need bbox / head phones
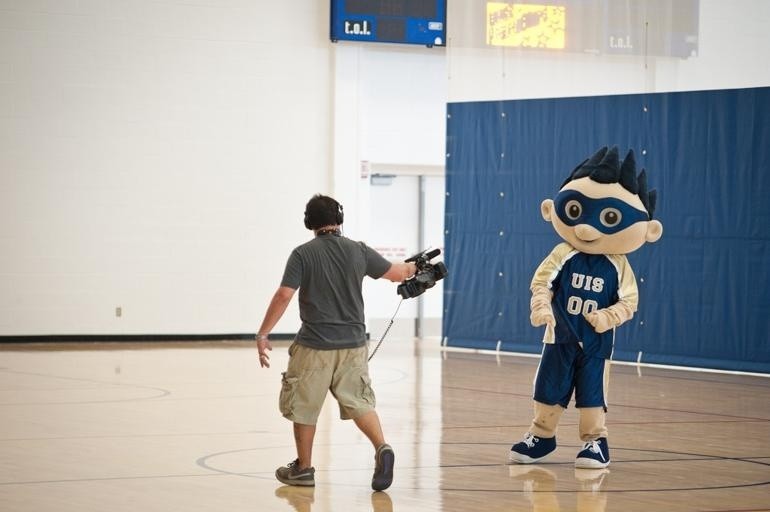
[304,203,344,230]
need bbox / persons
[275,485,393,512]
[255,192,419,491]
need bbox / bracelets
[256,336,268,339]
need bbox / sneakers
[371,443,395,491]
[275,460,316,486]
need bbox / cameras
[392,248,449,299]
